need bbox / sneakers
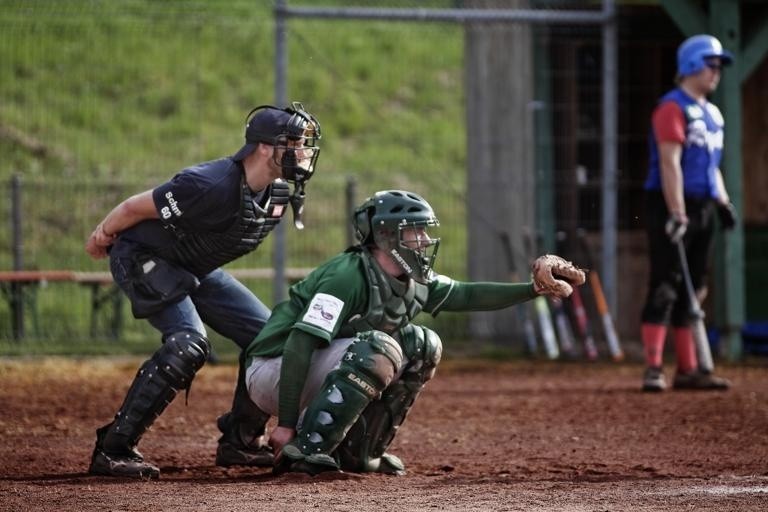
[214,438,274,467]
[91,428,160,481]
[674,370,731,388]
[642,365,667,389]
[276,448,407,476]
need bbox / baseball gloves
[531,252,587,304]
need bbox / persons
[243,189,585,474]
[85,101,321,481]
[640,34,737,391]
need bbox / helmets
[232,99,322,183]
[678,34,733,76]
[353,188,442,284]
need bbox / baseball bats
[496,227,625,366]
[670,214,715,377]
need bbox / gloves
[717,200,737,232]
[665,217,691,243]
